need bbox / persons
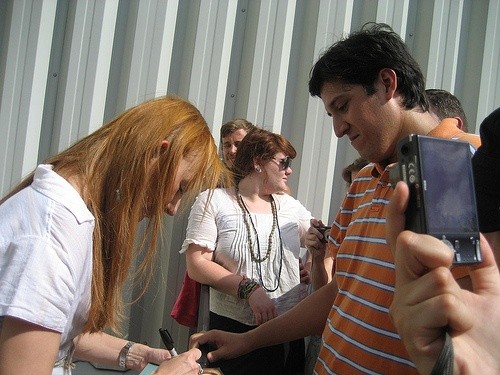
[0,96,204,375]
[180,127,315,375]
[341,90,500,375]
[220,120,293,196]
[188,22,482,375]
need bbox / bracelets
[118,341,135,372]
[236,276,259,299]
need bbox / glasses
[272,156,292,170]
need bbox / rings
[198,363,203,375]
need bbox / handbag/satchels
[170,270,200,327]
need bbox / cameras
[388,133,482,266]
[315,227,332,243]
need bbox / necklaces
[234,184,284,292]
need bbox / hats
[471,107,500,233]
